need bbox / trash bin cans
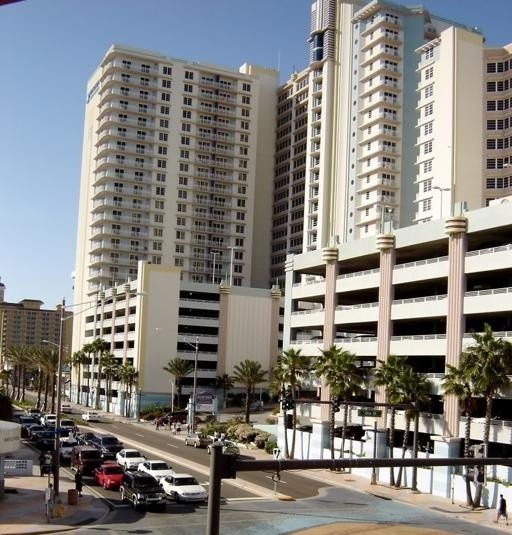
[68,489,78,505]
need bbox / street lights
[226,246,237,286]
[432,185,452,219]
[210,252,219,284]
[52,289,147,496]
[155,326,199,434]
[41,339,70,402]
[376,203,391,233]
[503,163,512,168]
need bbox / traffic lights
[283,390,295,410]
[334,396,340,412]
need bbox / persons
[50,483,55,518]
[38,450,52,476]
[75,469,84,497]
[154,414,191,436]
[493,494,508,526]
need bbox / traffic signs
[358,409,381,417]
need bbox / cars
[206,441,240,458]
[15,404,208,511]
[185,433,213,447]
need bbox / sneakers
[79,494,83,497]
[494,520,509,526]
